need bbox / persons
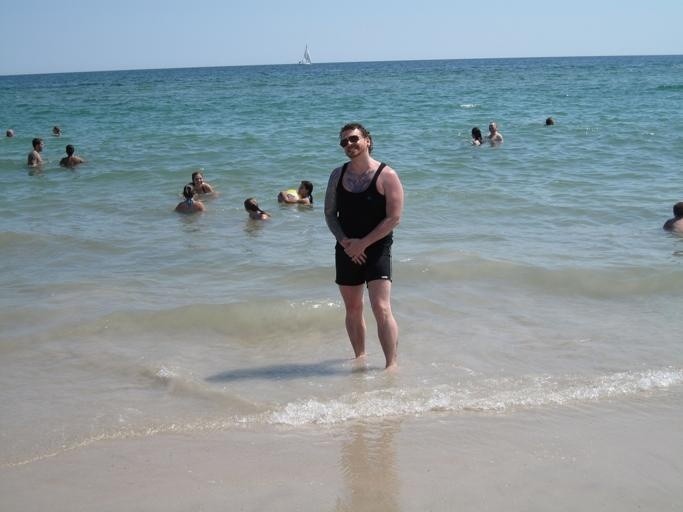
[488,122,502,144]
[663,202,683,231]
[279,181,313,205]
[471,127,483,146]
[191,172,212,194]
[6,129,14,137]
[176,185,204,212]
[244,198,270,219]
[546,116,555,124]
[324,124,404,368]
[60,145,84,166]
[53,126,61,136]
[27,138,43,165]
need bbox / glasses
[339,135,360,147]
